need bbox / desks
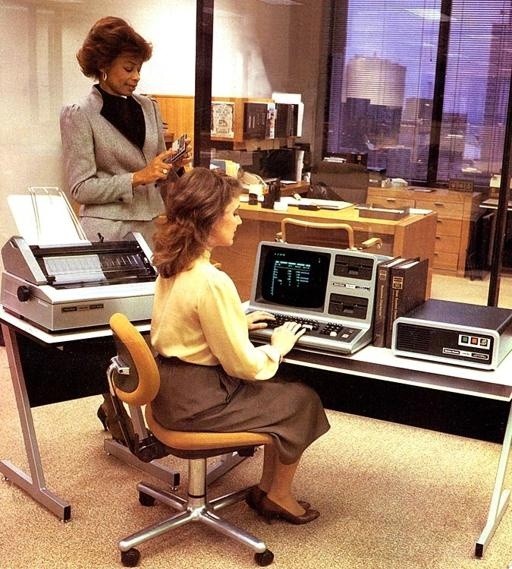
[204,200,436,311]
[0,308,512,557]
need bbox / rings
[162,168,168,175]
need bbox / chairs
[97,311,283,568]
[278,217,384,254]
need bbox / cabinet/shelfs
[209,96,312,198]
[364,187,482,276]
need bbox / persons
[58,14,193,435]
[139,165,339,526]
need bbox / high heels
[247,486,320,524]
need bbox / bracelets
[131,172,136,184]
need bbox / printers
[1,230,159,333]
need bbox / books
[207,90,313,199]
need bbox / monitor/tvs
[254,245,331,310]
